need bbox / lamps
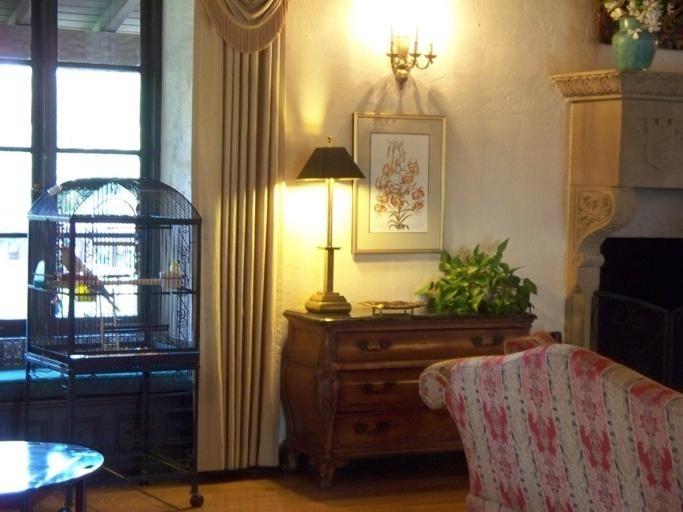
[386,14,438,82]
[296,136,366,313]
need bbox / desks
[0,438,104,511]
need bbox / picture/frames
[352,111,447,255]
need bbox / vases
[609,17,657,69]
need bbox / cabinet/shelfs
[275,310,539,493]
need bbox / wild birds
[56,243,122,312]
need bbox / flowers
[601,0,672,42]
